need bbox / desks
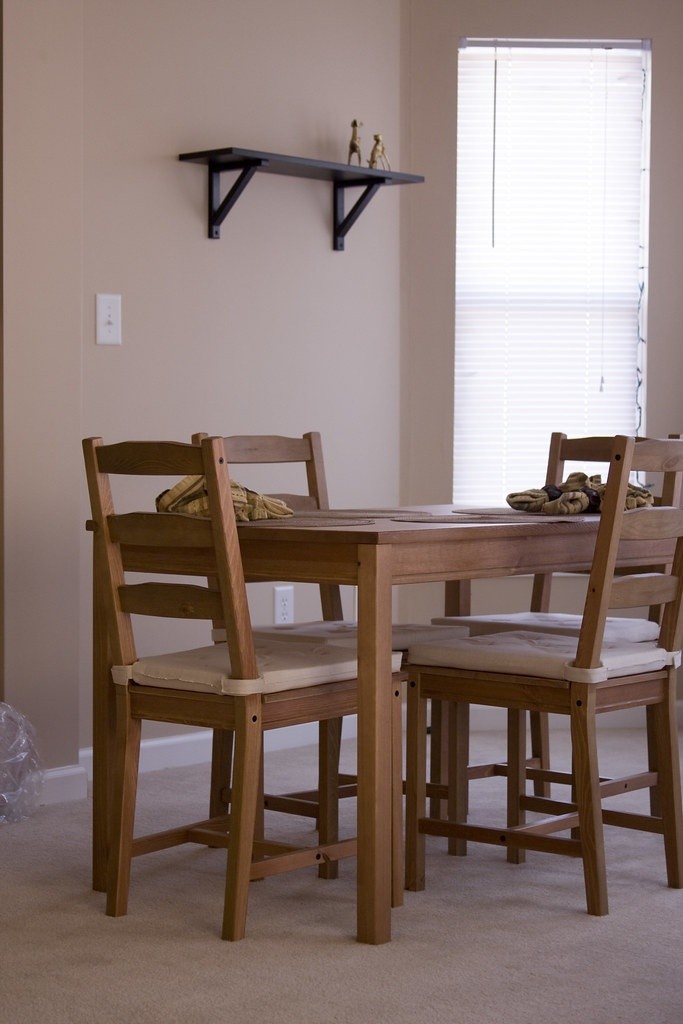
[81,507,678,945]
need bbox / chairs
[428,432,680,857]
[82,433,412,942]
[404,435,683,916]
[188,433,471,879]
